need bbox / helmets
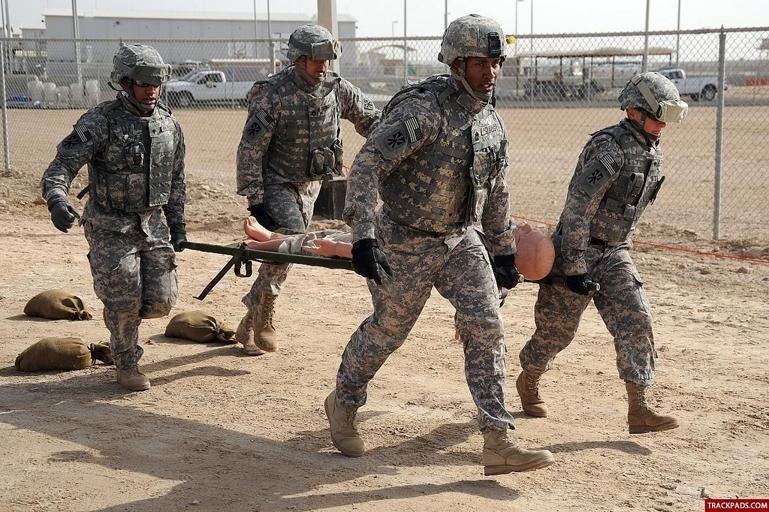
[287,24,342,63]
[437,13,508,65]
[618,72,688,122]
[109,42,171,87]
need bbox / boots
[235,311,264,354]
[324,389,364,457]
[626,382,679,433]
[482,431,554,476]
[252,291,278,352]
[515,364,547,418]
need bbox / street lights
[391,21,398,66]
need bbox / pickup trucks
[658,69,728,103]
[165,71,258,106]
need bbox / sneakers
[117,364,150,391]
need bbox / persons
[244,215,556,281]
[515,71,688,434]
[40,44,188,390]
[323,13,556,475]
[234,24,382,357]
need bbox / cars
[6,95,41,108]
[561,76,597,99]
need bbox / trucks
[168,59,282,84]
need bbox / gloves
[247,203,281,231]
[47,194,79,233]
[170,232,187,252]
[494,255,520,289]
[351,239,393,285]
[567,274,593,295]
